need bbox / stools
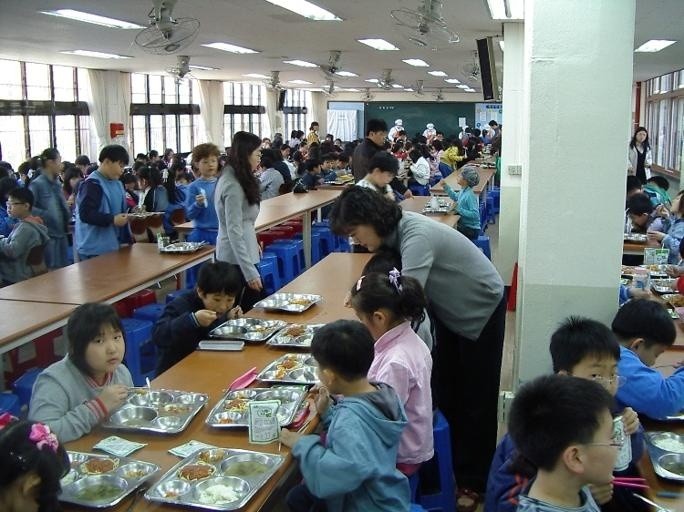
[479,202,488,231]
[487,198,495,223]
[265,243,301,284]
[127,289,157,309]
[0,392,22,417]
[112,300,134,317]
[261,252,277,266]
[256,260,281,293]
[294,233,321,265]
[12,367,46,411]
[166,288,192,306]
[260,231,287,245]
[134,302,168,322]
[274,239,306,272]
[507,262,518,311]
[492,190,499,213]
[121,318,157,386]
[409,410,457,512]
[270,226,295,239]
[313,222,329,227]
[282,221,302,232]
[312,227,334,253]
[470,236,491,260]
[289,215,303,220]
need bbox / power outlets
[517,165,522,174]
[508,165,517,174]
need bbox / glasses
[5,201,26,207]
[583,431,625,451]
[593,375,626,389]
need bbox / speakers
[477,37,500,101]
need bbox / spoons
[144,377,159,404]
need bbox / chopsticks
[290,418,318,437]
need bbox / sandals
[456,489,478,511]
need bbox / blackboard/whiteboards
[365,101,475,140]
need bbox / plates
[101,387,211,435]
[263,322,328,348]
[206,315,287,342]
[204,384,310,428]
[424,194,453,216]
[129,207,168,223]
[56,450,160,509]
[143,446,288,512]
[643,429,684,481]
[253,290,324,313]
[255,350,321,384]
[649,260,684,292]
[159,243,208,253]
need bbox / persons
[480,129,491,144]
[319,154,338,183]
[281,146,291,158]
[335,153,349,177]
[189,159,202,181]
[441,167,481,240]
[430,140,444,164]
[154,262,245,373]
[668,237,684,294]
[329,185,504,453]
[440,137,464,169]
[165,158,186,205]
[145,150,160,163]
[290,130,304,148]
[0,160,13,177]
[468,128,484,151]
[18,162,31,183]
[176,172,195,185]
[628,194,666,234]
[214,131,262,313]
[259,156,284,202]
[347,150,399,252]
[261,137,282,160]
[136,166,169,212]
[74,155,91,175]
[183,143,218,245]
[1,188,50,288]
[85,165,99,178]
[359,252,434,354]
[62,166,84,210]
[396,130,407,143]
[155,160,168,183]
[307,120,321,145]
[649,190,684,263]
[28,302,134,444]
[294,142,308,161]
[162,148,175,166]
[0,177,25,239]
[322,141,335,160]
[388,119,404,144]
[508,375,619,512]
[487,319,644,512]
[271,150,293,195]
[619,285,650,310]
[276,320,410,512]
[489,120,501,156]
[330,153,339,171]
[410,136,429,164]
[293,159,324,193]
[27,148,72,273]
[390,143,408,161]
[132,160,144,177]
[628,127,654,181]
[74,145,134,263]
[432,132,448,151]
[408,150,431,186]
[0,413,72,512]
[298,146,324,174]
[350,271,434,475]
[325,134,333,141]
[335,138,345,150]
[123,172,141,208]
[612,298,684,418]
[262,138,271,149]
[136,153,146,164]
[457,140,478,168]
[627,175,668,211]
[294,150,305,179]
[350,120,387,183]
[422,123,436,145]
[416,145,441,178]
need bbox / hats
[463,168,479,186]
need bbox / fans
[432,88,444,102]
[134,1,201,56]
[362,88,376,102]
[322,81,338,98]
[262,70,286,93]
[319,50,350,82]
[460,49,481,83]
[376,69,396,91]
[166,55,199,84]
[411,80,426,98]
[390,1,460,49]
